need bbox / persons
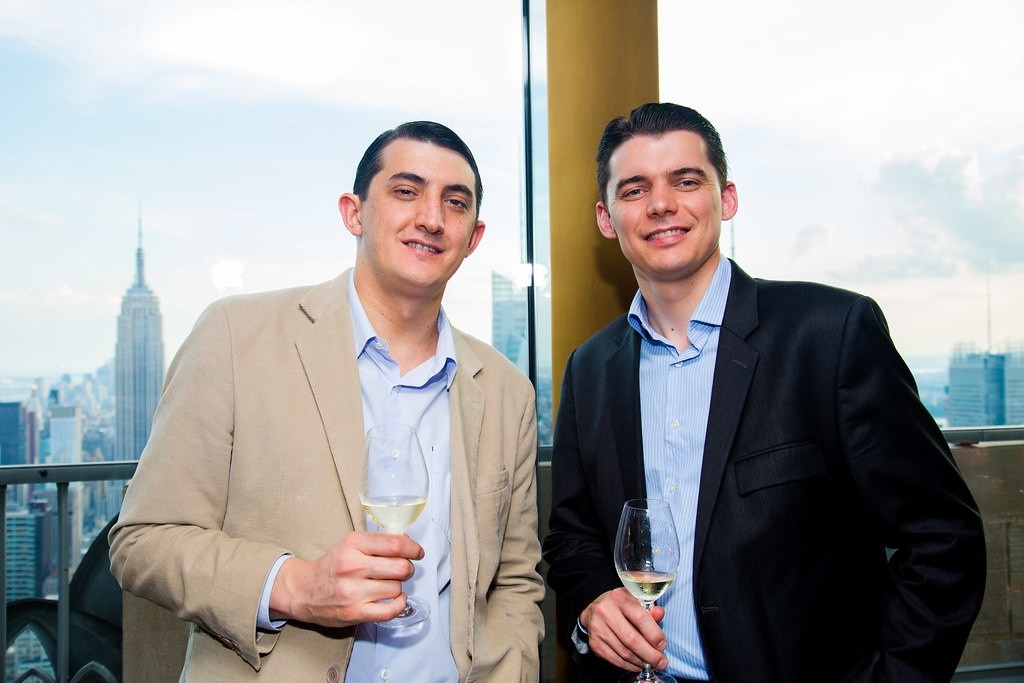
[540,102,987,683]
[106,120,545,683]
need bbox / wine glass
[359,427,434,628]
[612,498,682,683]
